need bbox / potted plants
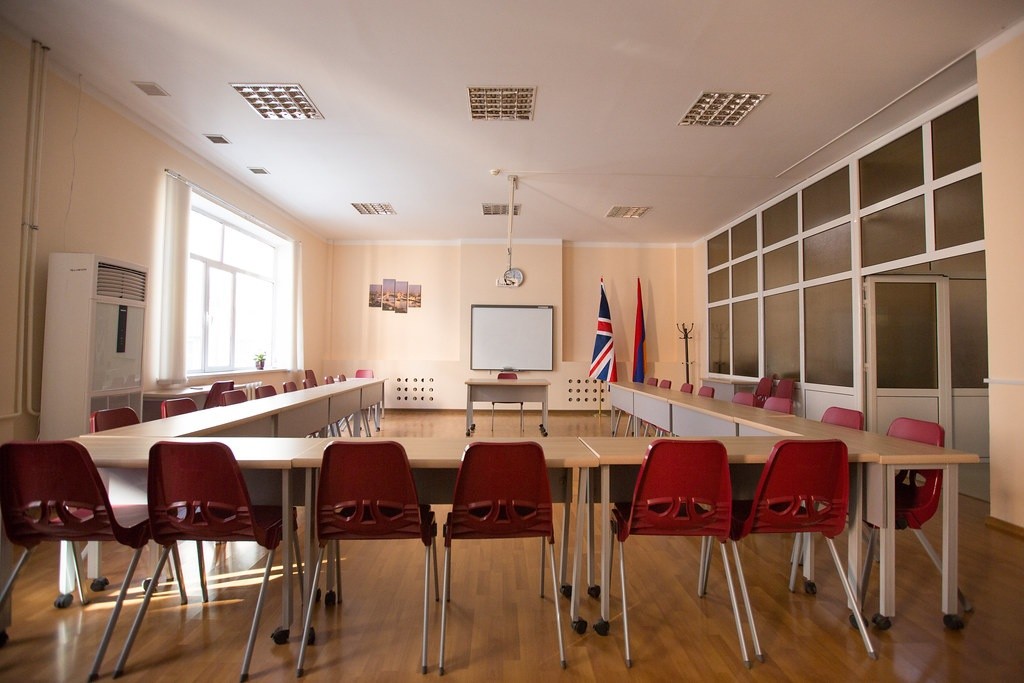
[252,353,266,370]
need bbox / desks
[59,392,980,560]
[141,384,249,423]
[293,437,600,645]
[463,378,550,436]
[607,382,657,436]
[333,377,377,384]
[58,436,321,643]
[700,378,758,404]
[338,377,388,433]
[579,436,882,633]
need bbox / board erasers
[502,367,512,371]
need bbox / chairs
[800,415,974,626]
[0,440,171,683]
[86,408,176,583]
[704,439,877,663]
[114,439,303,683]
[297,441,438,679]
[738,407,865,566]
[88,370,794,438]
[440,441,565,674]
[607,439,751,671]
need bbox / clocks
[504,269,524,287]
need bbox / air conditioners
[40,250,151,444]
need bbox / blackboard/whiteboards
[470,304,554,371]
